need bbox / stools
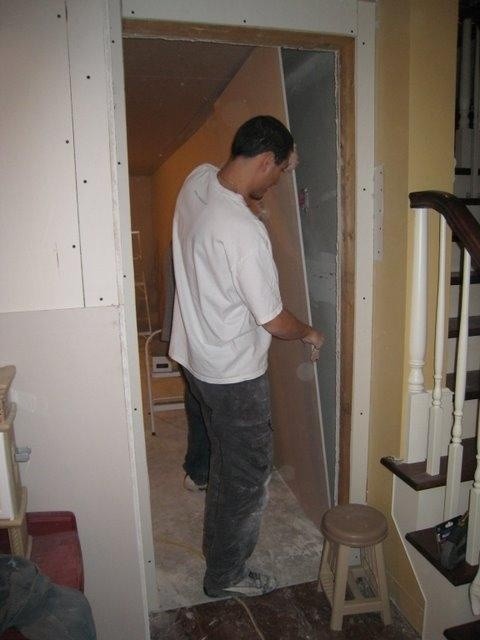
[314,503,393,632]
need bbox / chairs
[144,327,188,435]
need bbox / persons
[165,114,329,601]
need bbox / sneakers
[203,567,280,599]
[181,470,209,491]
